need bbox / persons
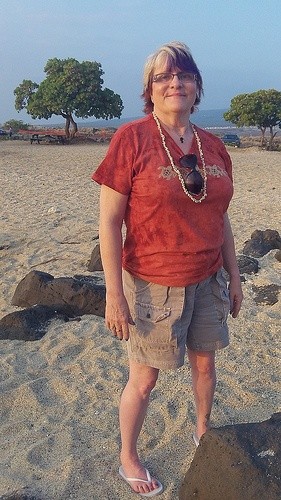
[90,40,244,499]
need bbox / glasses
[179,154,202,195]
[153,72,197,82]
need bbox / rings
[115,329,122,333]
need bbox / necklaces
[152,112,208,204]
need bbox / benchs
[30,139,66,145]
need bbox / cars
[0,129,24,140]
[221,134,241,147]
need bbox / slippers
[193,433,200,447]
[119,464,163,496]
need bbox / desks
[32,134,66,144]
